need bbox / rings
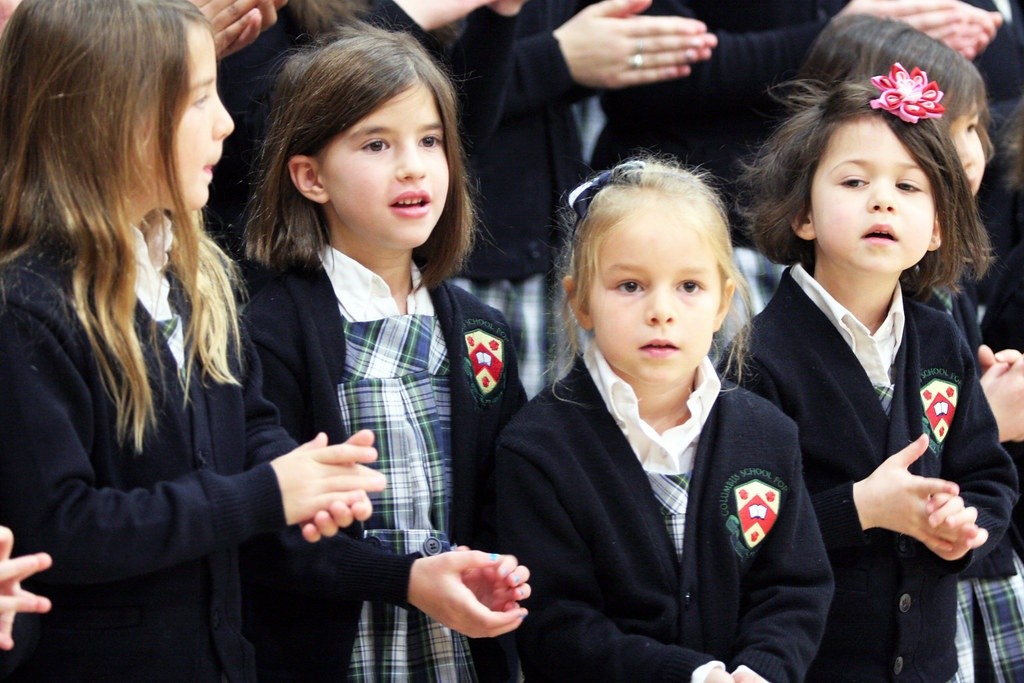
[629,52,644,69]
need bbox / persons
[490,150,837,683]
[783,11,1023,681]
[194,2,1023,404]
[236,25,528,683]
[0,1,389,683]
[724,58,1020,681]
[0,523,51,652]
